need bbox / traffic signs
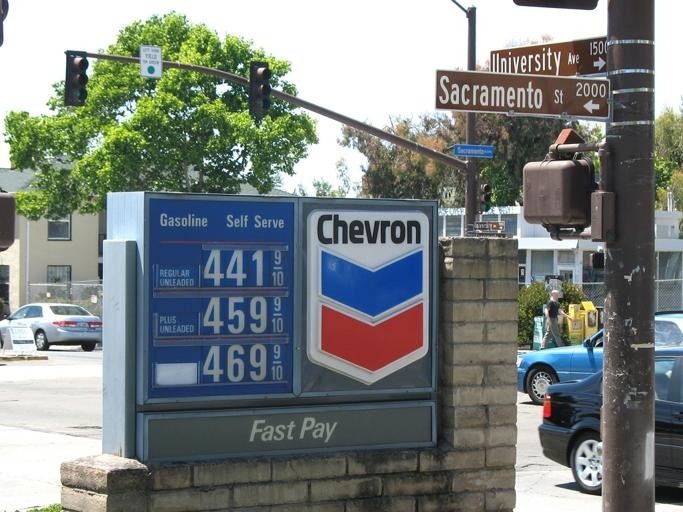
[477,232,506,238]
[139,45,163,79]
[454,144,495,158]
[435,70,609,125]
[487,36,607,78]
[473,220,505,230]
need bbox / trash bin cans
[533,301,603,350]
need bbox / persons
[539,290,573,349]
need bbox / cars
[515,310,682,406]
[0,303,102,351]
[539,346,682,498]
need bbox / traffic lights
[247,60,271,117]
[593,254,604,269]
[480,184,491,213]
[64,52,88,106]
